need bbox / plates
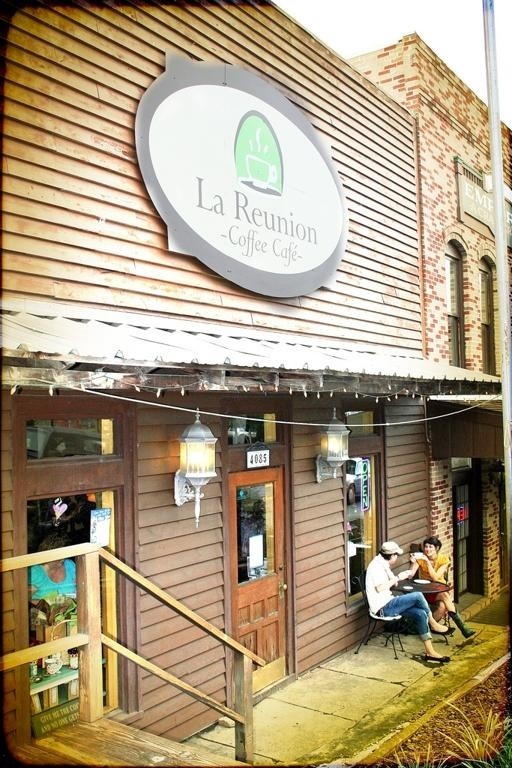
[413,579,431,584]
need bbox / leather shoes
[424,655,450,662]
[431,627,455,635]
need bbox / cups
[44,659,63,675]
[411,552,424,560]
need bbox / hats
[382,541,404,555]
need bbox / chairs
[351,550,455,660]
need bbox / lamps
[174,406,218,529]
[320,406,351,479]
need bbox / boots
[448,609,475,638]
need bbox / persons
[26,533,80,603]
[408,536,476,638]
[365,540,454,662]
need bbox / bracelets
[395,575,401,581]
[425,559,429,563]
